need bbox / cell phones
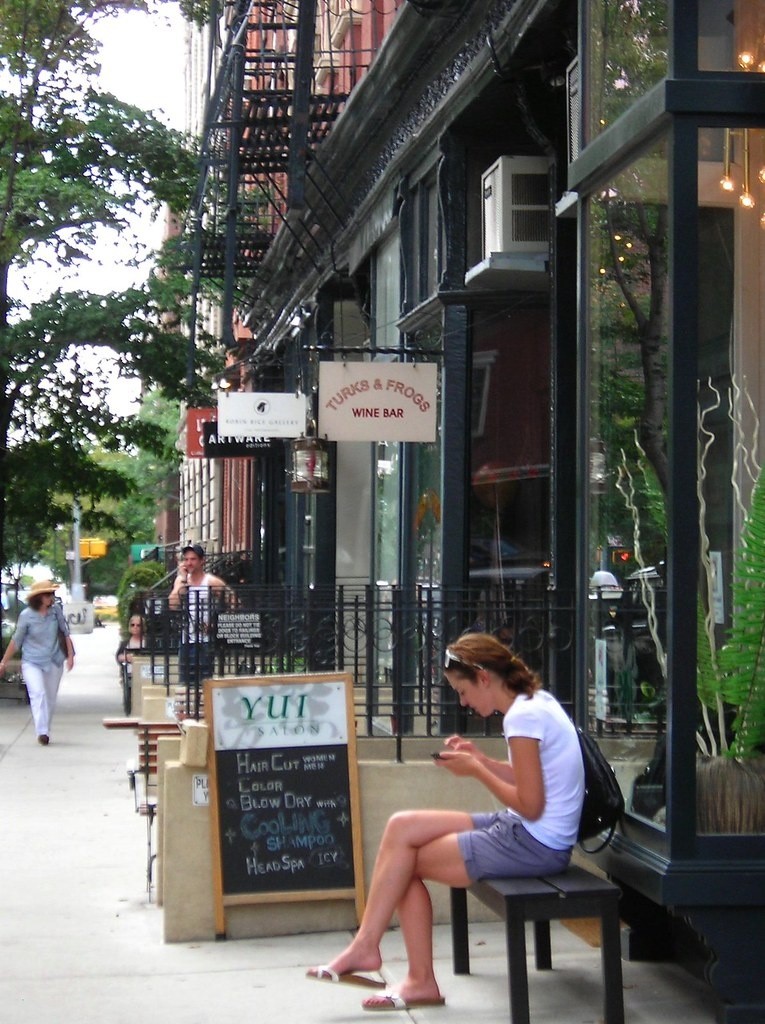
[431,752,444,761]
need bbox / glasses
[444,649,484,670]
[130,624,140,628]
[37,592,55,597]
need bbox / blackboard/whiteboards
[199,673,368,909]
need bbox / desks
[101,717,220,902]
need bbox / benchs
[451,864,626,1024]
[127,721,204,902]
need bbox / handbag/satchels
[574,722,625,854]
[53,605,75,658]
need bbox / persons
[306,632,585,1013]
[168,545,242,689]
[0,579,76,745]
[94,614,103,627]
[115,615,150,716]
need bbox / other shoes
[36,734,50,746]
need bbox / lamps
[737,50,765,72]
[719,128,765,231]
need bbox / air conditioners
[481,156,558,261]
[566,55,579,167]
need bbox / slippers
[304,964,386,984]
[361,989,446,1009]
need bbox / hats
[181,543,204,557]
[25,579,60,601]
